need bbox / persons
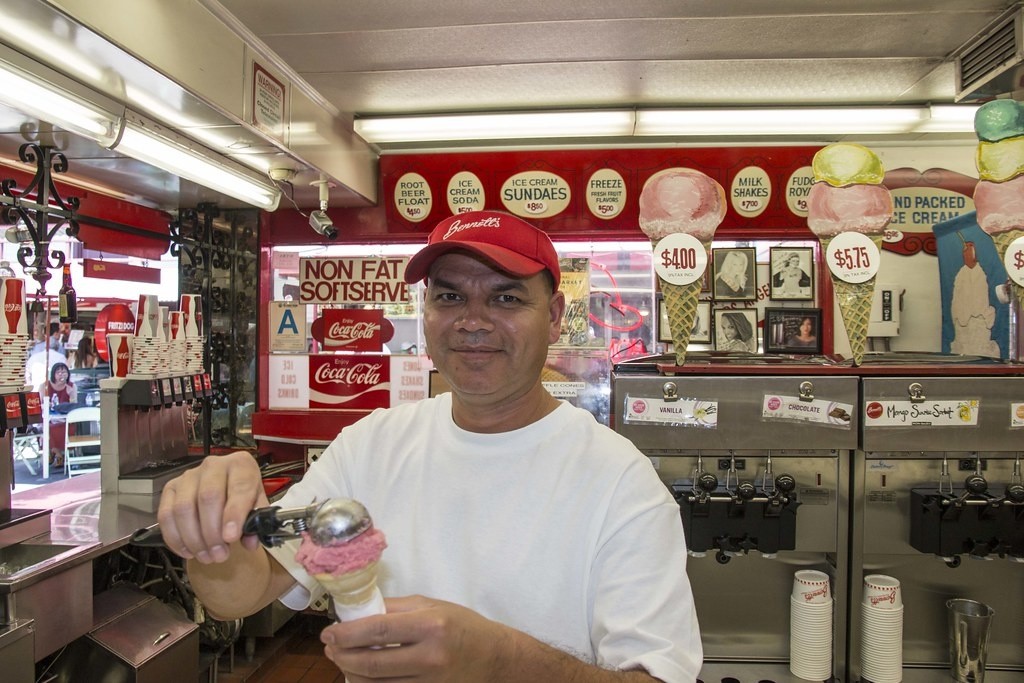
[788,317,816,346]
[773,252,811,295]
[718,313,754,352]
[24,323,99,469]
[156,211,703,683]
[715,250,748,296]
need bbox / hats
[404,210,560,295]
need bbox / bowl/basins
[860,574,903,683]
[789,570,833,681]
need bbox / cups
[946,598,995,683]
[104,293,204,379]
[0,277,31,394]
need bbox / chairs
[65,406,102,478]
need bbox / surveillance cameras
[309,210,337,240]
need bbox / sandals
[55,454,64,467]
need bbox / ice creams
[294,522,388,608]
[639,167,727,365]
[807,142,893,367]
[973,99,1024,309]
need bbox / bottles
[58,263,79,324]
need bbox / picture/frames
[712,309,757,353]
[657,298,712,344]
[764,307,823,353]
[712,247,758,300]
[770,247,814,299]
[651,243,711,293]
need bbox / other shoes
[49,451,56,464]
